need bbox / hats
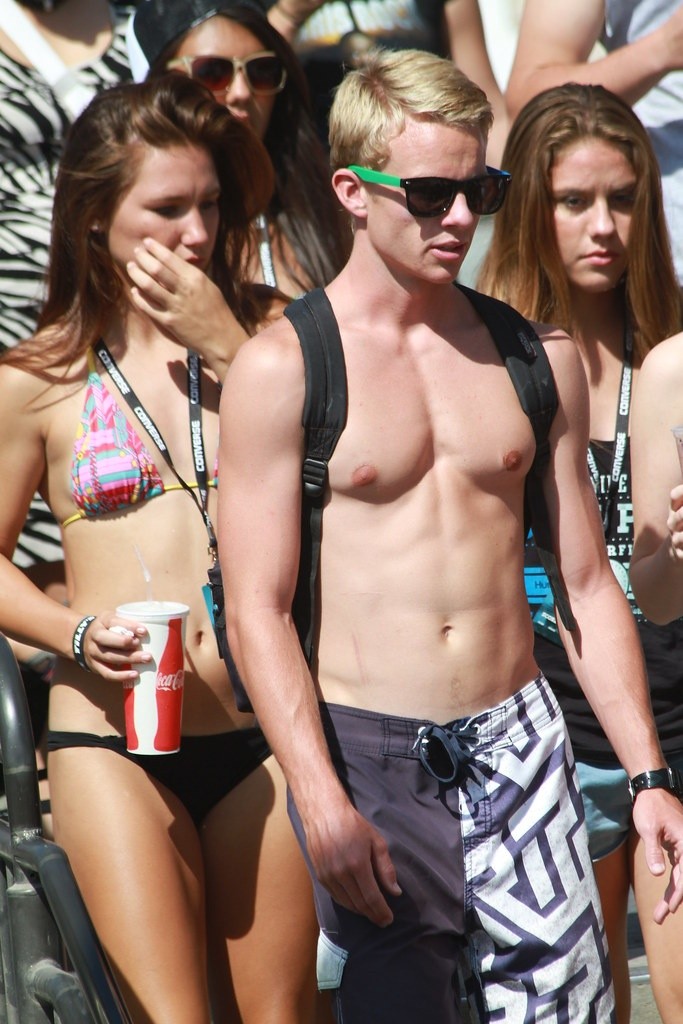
[133,0,227,82]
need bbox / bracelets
[73,616,97,675]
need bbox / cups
[115,600,190,756]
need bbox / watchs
[627,766,683,806]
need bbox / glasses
[348,165,511,219]
[167,52,288,96]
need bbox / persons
[0,0,683,1024]
